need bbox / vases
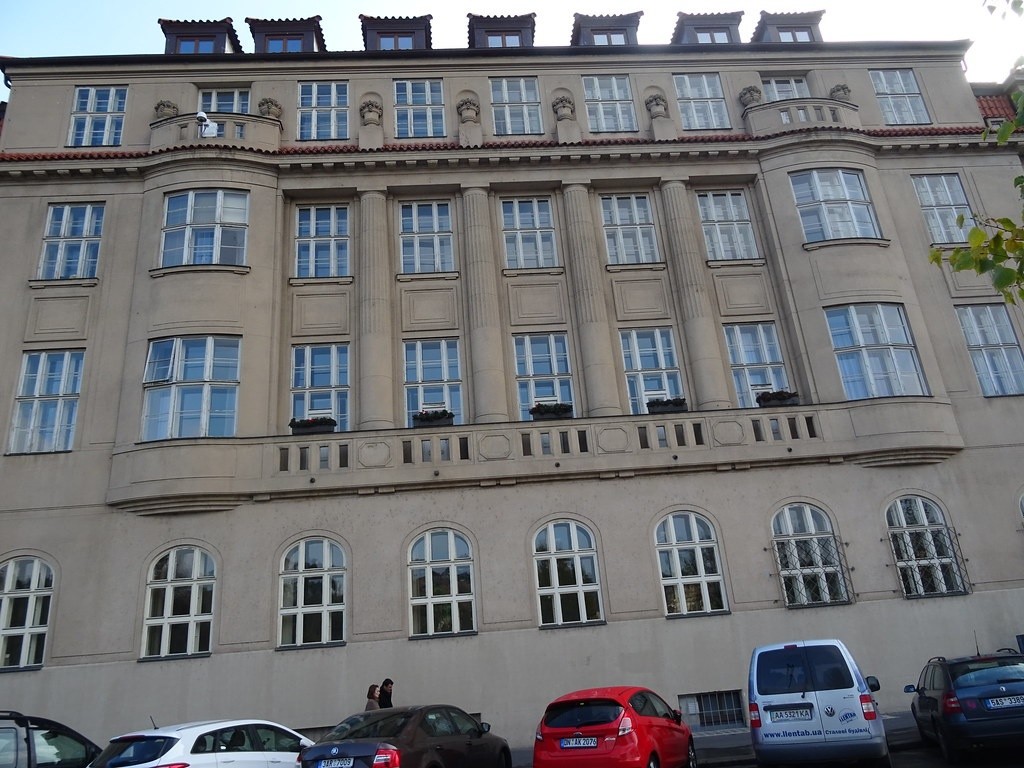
[647,403,688,414]
[531,412,574,420]
[290,425,335,434]
[412,418,454,427]
[758,396,799,407]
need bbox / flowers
[756,390,799,403]
[412,409,455,419]
[529,401,574,415]
[288,415,338,427]
[645,396,685,406]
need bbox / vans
[746,638,892,767]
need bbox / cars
[532,687,699,768]
[904,646,1024,768]
[299,704,512,767]
[0,710,102,768]
[83,720,317,768]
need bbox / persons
[363,683,380,712]
[377,677,395,708]
